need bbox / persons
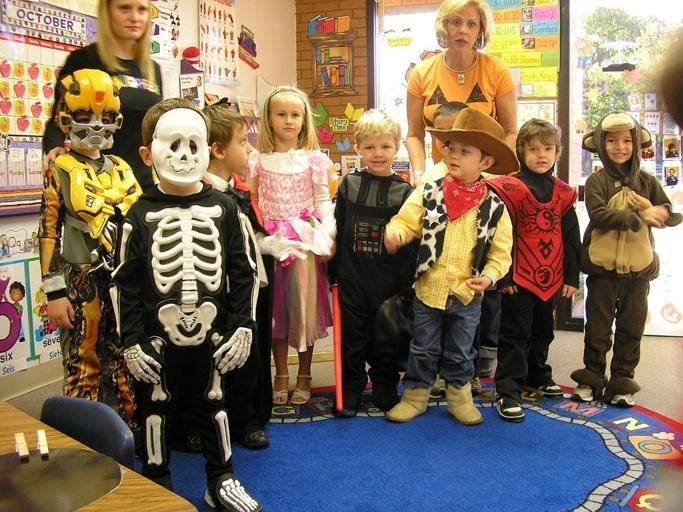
[569,109,680,409]
[198,98,308,449]
[323,107,418,421]
[486,118,566,425]
[35,69,151,462]
[641,137,653,159]
[665,168,678,185]
[405,0,518,193]
[110,98,267,512]
[244,85,338,408]
[384,105,516,434]
[42,0,165,185]
[426,100,473,177]
[664,142,678,158]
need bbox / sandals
[272,373,290,405]
[291,374,312,405]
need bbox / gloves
[392,280,414,316]
[254,224,310,263]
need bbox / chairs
[38,395,135,471]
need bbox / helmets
[54,68,123,151]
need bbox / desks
[0,399,200,511]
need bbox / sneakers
[611,393,634,407]
[477,358,491,377]
[182,433,202,453]
[470,376,482,395]
[241,428,269,450]
[203,471,261,512]
[495,393,524,422]
[524,383,563,400]
[572,384,593,402]
[430,374,445,398]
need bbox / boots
[385,386,431,422]
[445,380,483,425]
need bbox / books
[306,11,355,95]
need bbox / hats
[424,107,521,175]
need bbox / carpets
[171,373,681,512]
